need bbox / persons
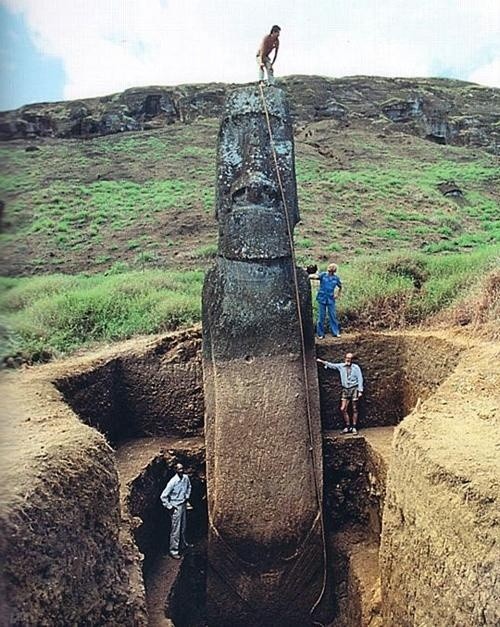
[311,348,365,436]
[157,460,195,560]
[306,262,347,340]
[252,22,282,82]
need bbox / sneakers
[183,542,194,548]
[319,333,341,339]
[168,551,181,559]
[343,426,357,432]
